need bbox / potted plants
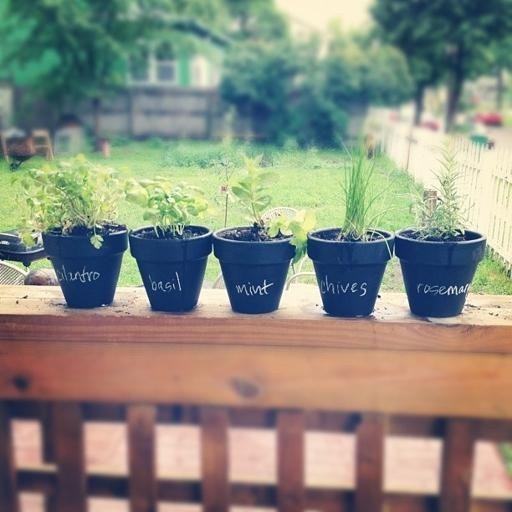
[396,153,485,317]
[306,148,396,316]
[16,154,129,308]
[217,149,297,315]
[128,173,213,311]
